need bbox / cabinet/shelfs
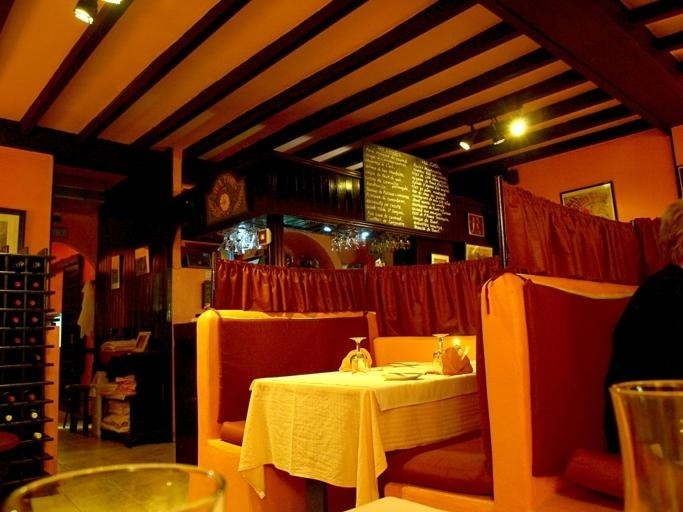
[0,245,56,502]
[100,351,169,449]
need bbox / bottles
[287,255,320,268]
[217,231,259,260]
[4,258,43,440]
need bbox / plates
[401,361,416,366]
[383,372,425,381]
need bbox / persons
[602,197,683,455]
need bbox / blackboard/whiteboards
[363,142,457,242]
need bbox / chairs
[63,349,100,436]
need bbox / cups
[7,462,226,511]
[609,378,683,512]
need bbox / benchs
[499,273,639,512]
[383,277,499,512]
[194,309,390,511]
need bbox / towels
[339,348,373,372]
[442,348,473,374]
[96,338,137,434]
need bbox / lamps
[490,117,506,146]
[459,127,480,151]
[73,0,98,25]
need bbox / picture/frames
[675,165,683,198]
[464,240,494,261]
[430,251,450,265]
[0,207,28,252]
[559,178,619,223]
[134,331,152,353]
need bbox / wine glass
[348,337,370,373]
[433,334,456,372]
[330,229,411,252]
